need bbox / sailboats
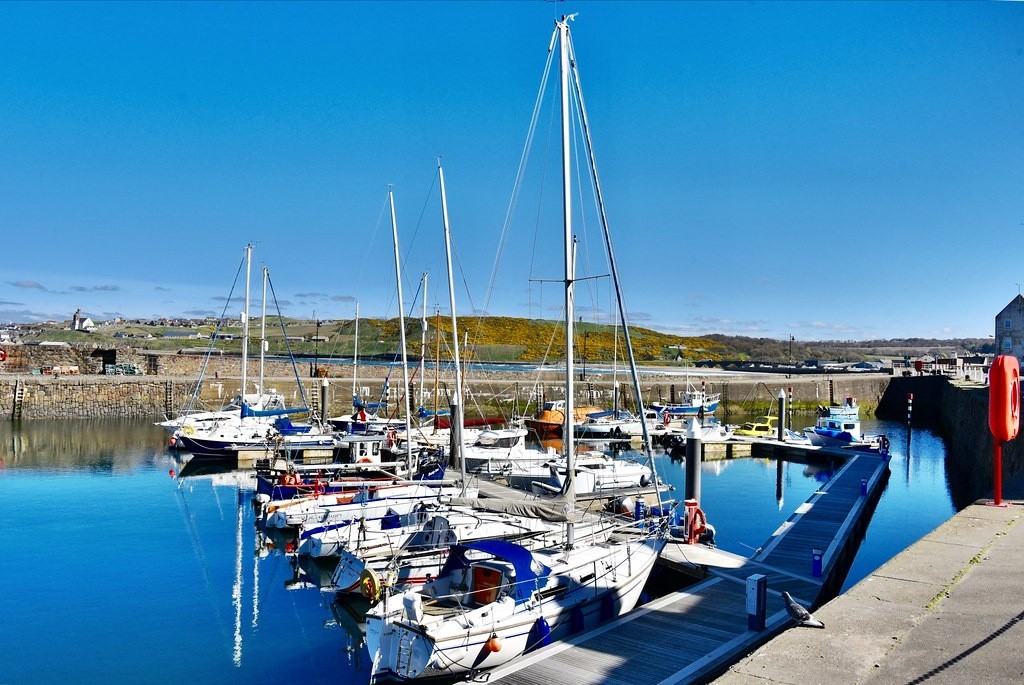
[151,5,879,681]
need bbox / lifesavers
[880,438,889,449]
[691,508,706,533]
[387,431,396,447]
[184,426,194,434]
[359,458,373,463]
[360,568,381,600]
